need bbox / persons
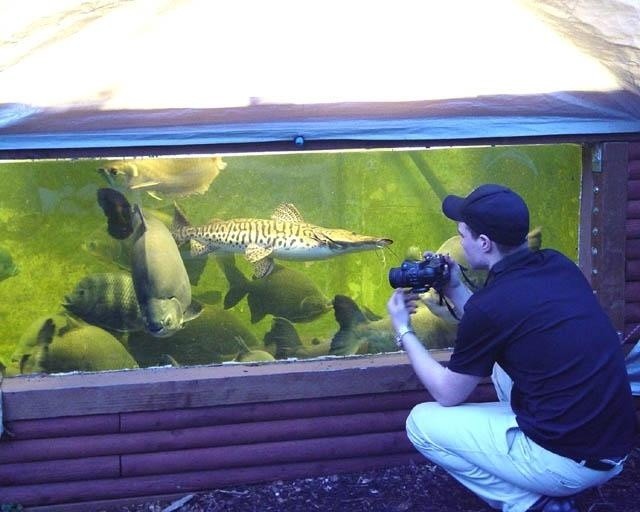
[385,184,633,512]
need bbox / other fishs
[10,199,401,377]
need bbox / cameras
[388,253,451,293]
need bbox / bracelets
[398,326,415,338]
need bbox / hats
[442,183,530,245]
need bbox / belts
[572,458,615,471]
